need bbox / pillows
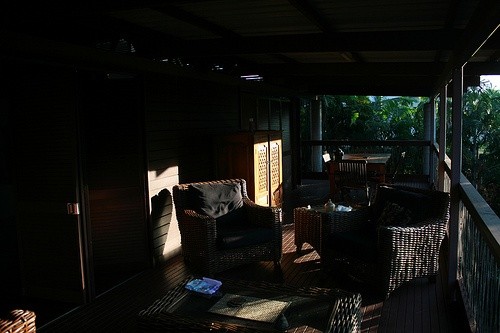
[186,180,247,229]
[375,199,416,228]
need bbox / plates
[227,295,247,307]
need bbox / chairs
[334,147,401,207]
[172,177,283,276]
[365,185,452,298]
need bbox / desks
[137,271,364,333]
[294,204,362,271]
[339,153,392,183]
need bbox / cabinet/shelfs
[228,127,284,209]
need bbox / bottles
[324,198,335,212]
